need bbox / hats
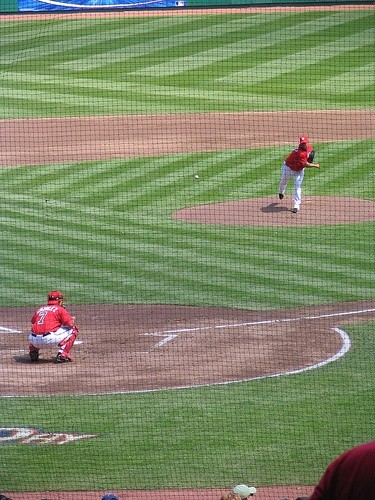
[300,136,307,144]
[47,291,62,304]
[102,493,118,500]
[234,484,256,499]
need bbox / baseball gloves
[307,151,315,163]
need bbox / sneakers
[56,355,72,363]
[29,351,39,361]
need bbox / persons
[28,291,79,361]
[234,485,256,500]
[278,136,320,213]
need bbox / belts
[32,332,50,337]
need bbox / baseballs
[195,175,199,179]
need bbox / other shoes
[279,193,283,199]
[293,208,297,213]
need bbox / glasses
[249,494,253,496]
